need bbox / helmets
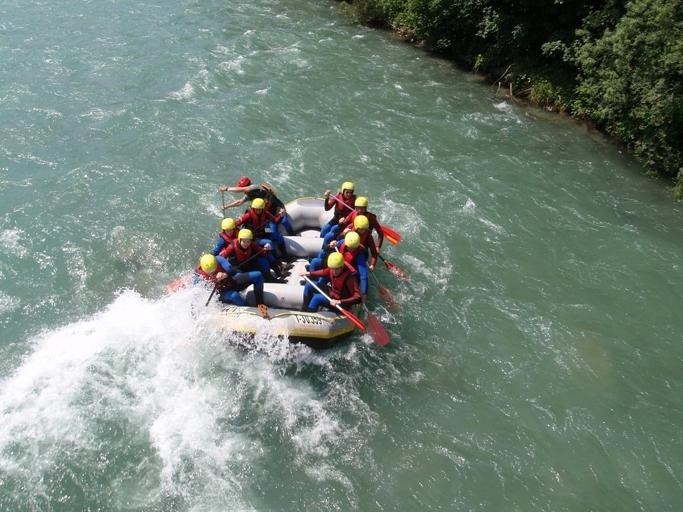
[327,182,369,269]
[200,177,265,274]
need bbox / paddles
[335,247,389,346]
[378,253,405,277]
[302,275,366,331]
[366,262,401,314]
[329,194,400,245]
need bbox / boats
[221,198,361,348]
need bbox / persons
[300,181,383,312]
[167,177,296,306]
